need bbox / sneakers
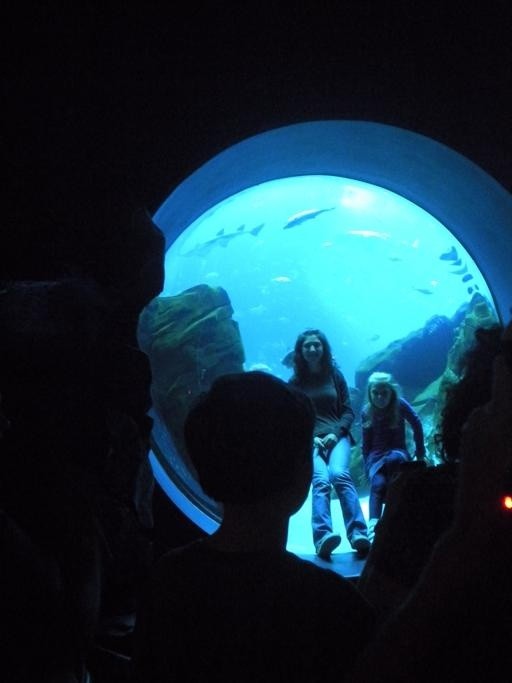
[315,533,341,556]
[352,534,371,555]
